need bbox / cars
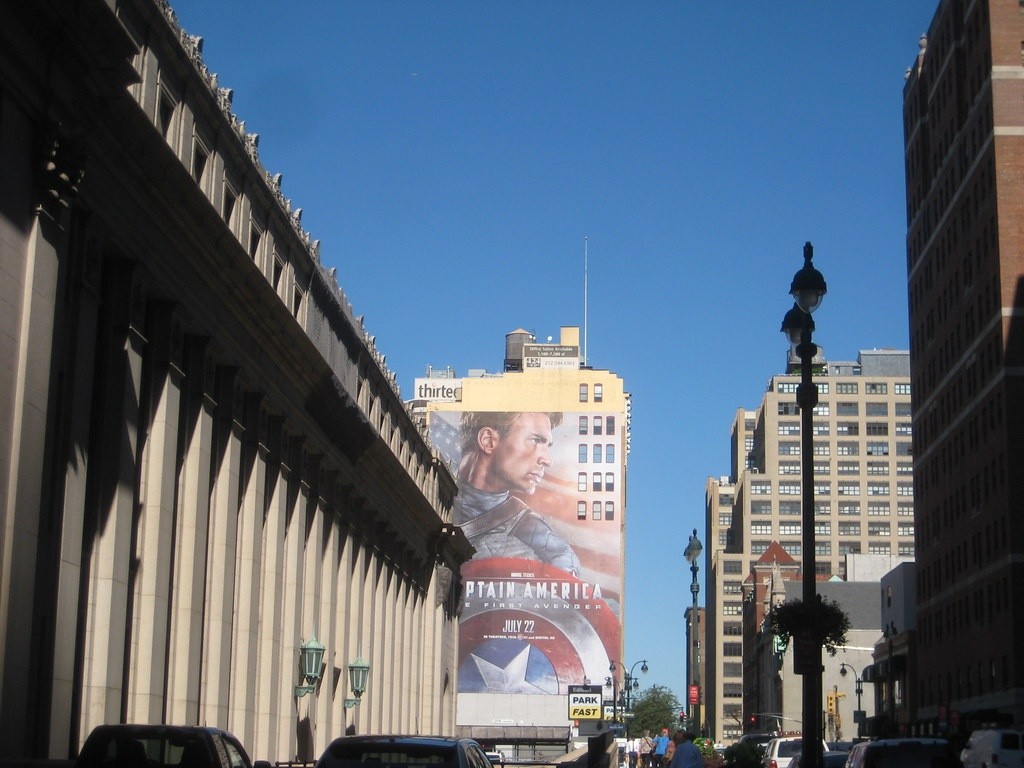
[74,724,272,768]
[313,733,494,768]
[715,727,1024,768]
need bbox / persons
[435,410,592,607]
[612,720,710,768]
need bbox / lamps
[294,637,327,696]
[343,659,371,707]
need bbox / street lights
[681,527,704,740]
[838,662,864,739]
[778,240,829,768]
[606,656,649,742]
